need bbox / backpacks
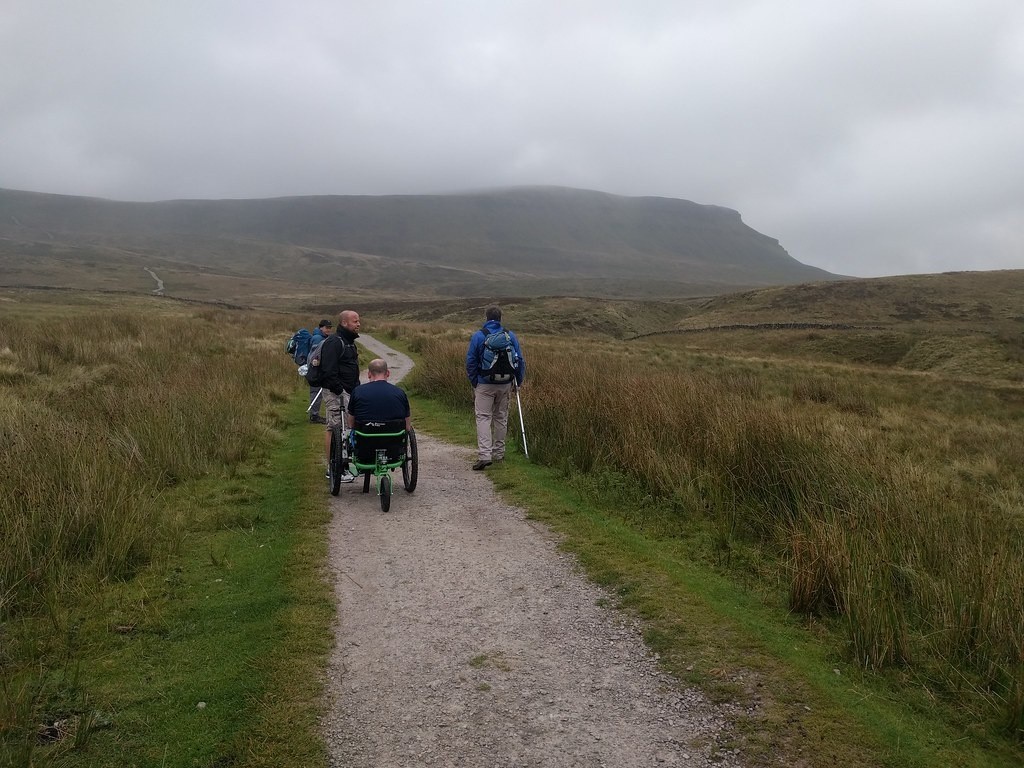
[305,336,345,387]
[287,329,313,366]
[477,327,519,385]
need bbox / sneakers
[325,464,354,483]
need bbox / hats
[319,320,332,329]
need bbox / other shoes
[310,415,326,424]
[473,460,493,470]
[496,457,504,463]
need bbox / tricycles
[328,395,418,512]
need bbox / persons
[465,308,525,470]
[320,310,360,483]
[305,319,332,424]
[347,359,411,432]
